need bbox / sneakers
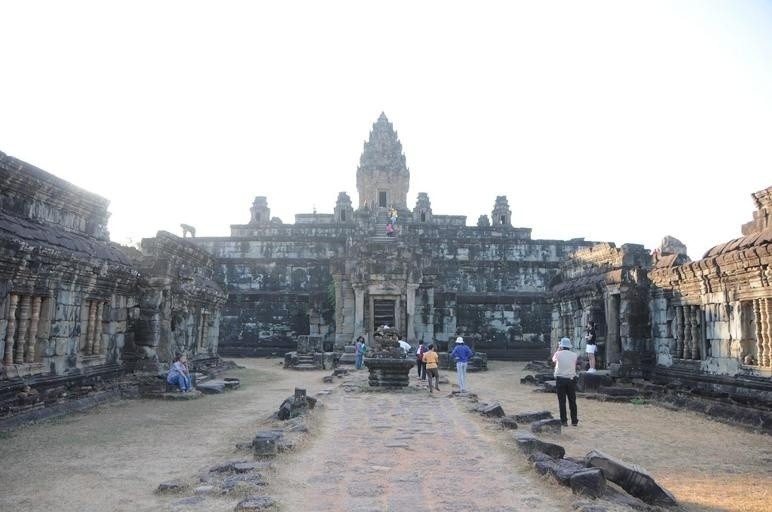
[589,368,596,373]
[586,368,591,373]
[560,420,568,426]
[572,419,578,425]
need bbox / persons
[354,335,366,371]
[167,349,197,395]
[551,337,579,427]
[384,205,400,237]
[452,335,474,393]
[377,322,387,332]
[423,344,442,393]
[584,315,597,375]
[416,339,425,382]
[399,335,412,357]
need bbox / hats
[559,337,572,348]
[455,336,464,343]
